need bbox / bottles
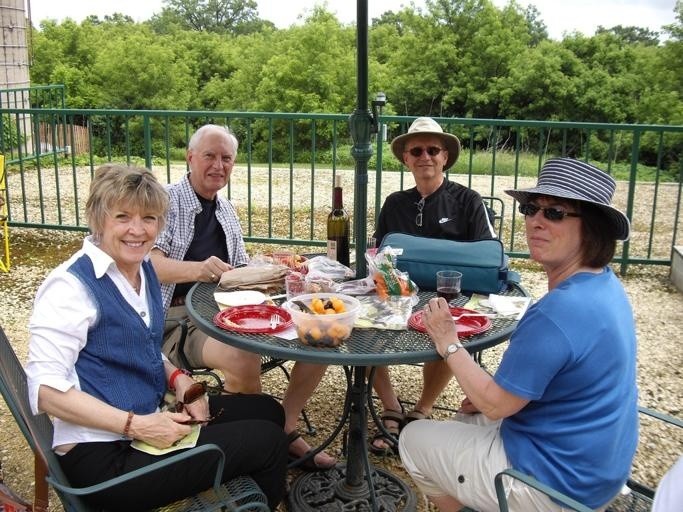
[326,187,350,269]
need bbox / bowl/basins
[286,293,360,348]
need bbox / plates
[408,307,492,336]
[213,304,294,334]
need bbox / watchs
[443,342,465,365]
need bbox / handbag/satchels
[376,232,520,295]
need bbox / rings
[210,273,215,281]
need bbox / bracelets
[122,409,134,437]
[167,367,191,393]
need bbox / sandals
[368,404,404,455]
[286,429,336,472]
[399,410,425,432]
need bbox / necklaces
[131,282,141,292]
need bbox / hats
[505,157,628,241]
[391,116,460,171]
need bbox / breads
[373,272,413,299]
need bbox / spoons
[451,311,496,321]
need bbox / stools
[178,350,317,435]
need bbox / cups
[285,274,311,300]
[436,270,462,303]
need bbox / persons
[343,117,500,458]
[397,156,640,512]
[150,123,334,470]
[24,164,290,511]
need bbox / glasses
[168,381,240,425]
[518,204,583,220]
[414,198,425,226]
[403,146,444,157]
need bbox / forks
[270,313,280,330]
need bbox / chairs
[0,326,271,512]
[456,406,682,512]
[368,196,505,422]
